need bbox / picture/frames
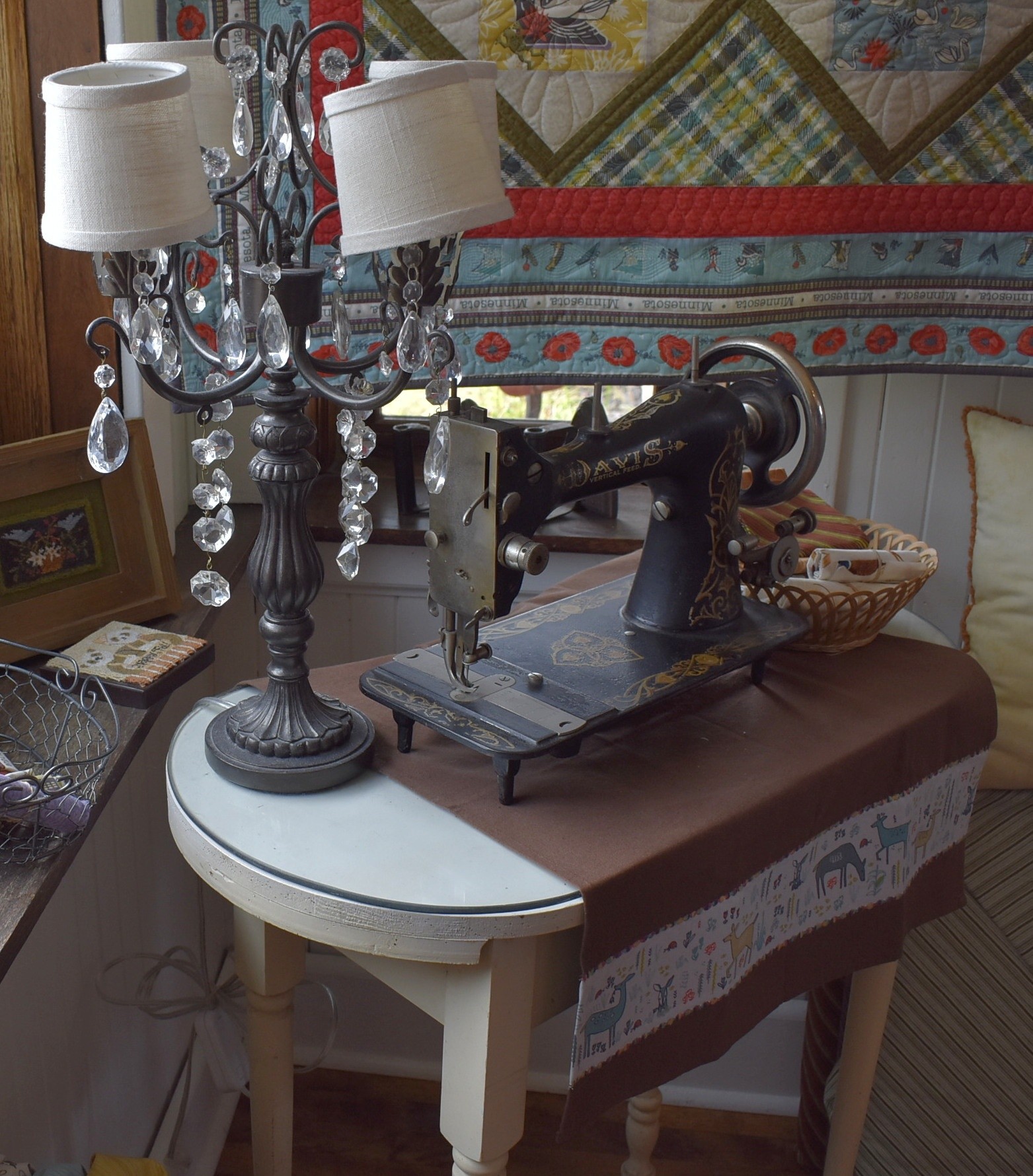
[0,419,183,665]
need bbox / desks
[165,579,1002,1176]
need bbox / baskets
[738,468,938,651]
[0,638,119,865]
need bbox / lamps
[39,18,513,792]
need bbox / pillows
[956,406,1033,789]
